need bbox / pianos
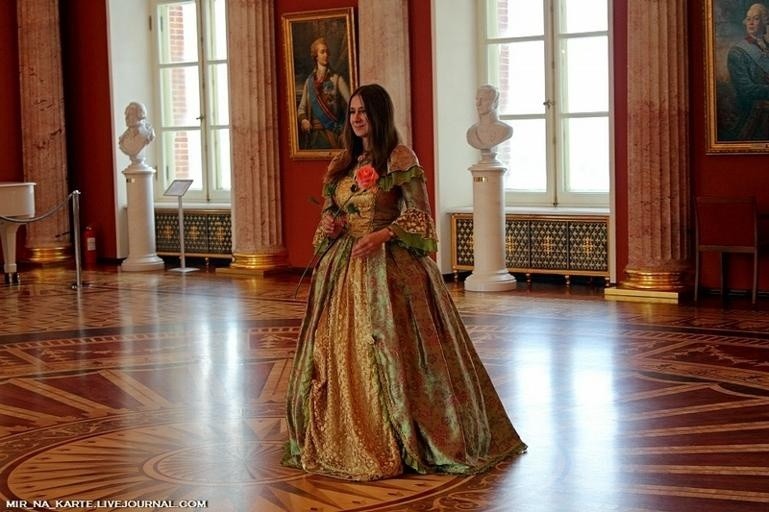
[0,183,38,284]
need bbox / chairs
[692,193,769,307]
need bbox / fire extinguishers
[81,224,96,266]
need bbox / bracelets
[386,226,395,241]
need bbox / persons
[118,102,155,157]
[283,84,525,476]
[295,36,349,149]
[719,3,769,140]
[466,83,514,149]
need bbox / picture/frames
[703,0,769,158]
[281,5,365,164]
